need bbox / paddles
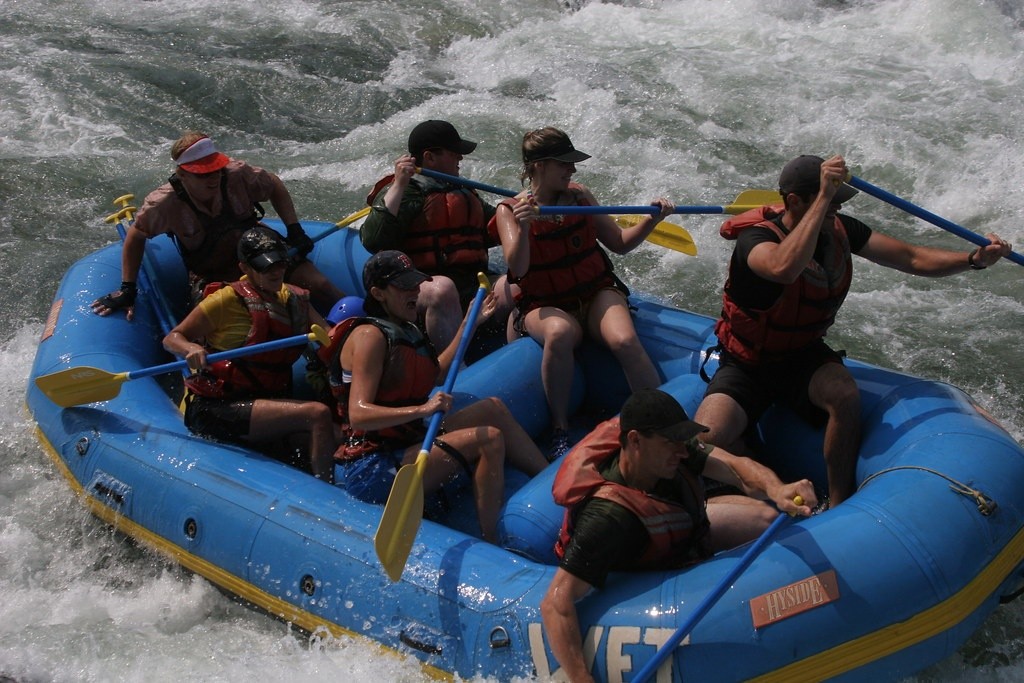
[238,205,369,282]
[36,324,332,409]
[105,194,195,417]
[632,495,801,683]
[415,164,696,255]
[843,168,1023,265]
[535,189,783,215]
[374,271,492,581]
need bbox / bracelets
[968,249,987,270]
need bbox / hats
[620,388,710,442]
[523,140,592,165]
[237,227,290,272]
[408,120,478,155]
[363,250,433,291]
[178,136,230,174]
[779,155,859,205]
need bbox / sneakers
[551,428,571,461]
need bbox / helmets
[326,295,368,326]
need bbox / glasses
[423,146,462,157]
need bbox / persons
[693,153,1012,508]
[92,133,339,321]
[540,388,817,683]
[359,119,522,386]
[318,252,551,544]
[163,224,342,483]
[496,127,675,462]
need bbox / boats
[25,216,1024,683]
